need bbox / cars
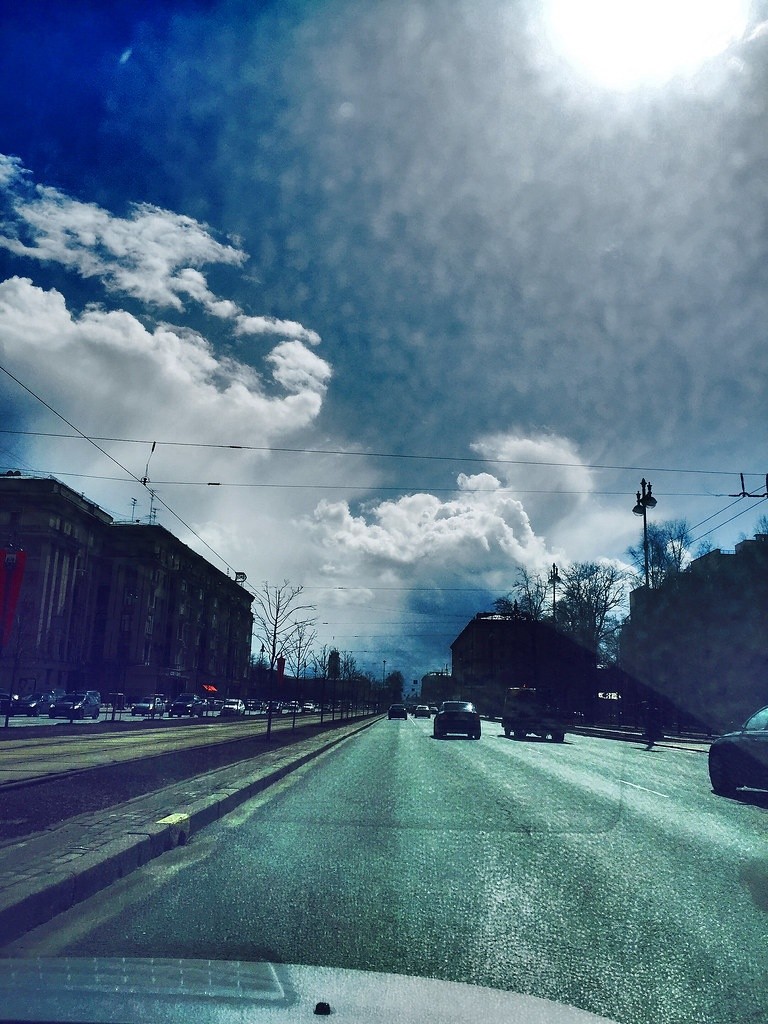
[0,690,330,717]
[414,705,431,719]
[49,692,101,720]
[407,704,438,714]
[130,696,166,716]
[387,704,408,720]
[708,705,768,796]
[433,700,482,740]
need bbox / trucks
[501,684,569,742]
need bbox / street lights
[547,564,561,681]
[631,479,666,740]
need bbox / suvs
[169,694,203,717]
[220,699,246,716]
